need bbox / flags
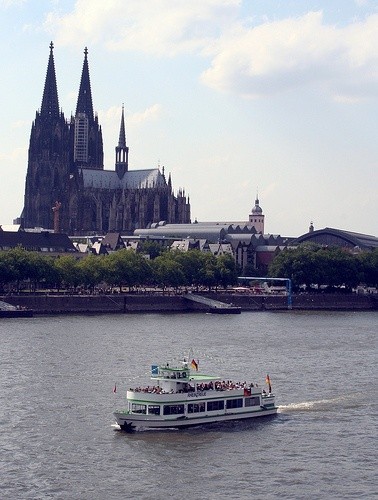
[266,374,271,385]
[191,359,197,370]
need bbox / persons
[16,303,29,310]
[66,286,118,296]
[5,288,26,296]
[130,385,162,394]
[164,405,184,415]
[188,404,205,413]
[149,408,160,415]
[169,380,258,394]
[132,284,232,296]
[235,288,341,296]
[263,389,271,397]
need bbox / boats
[111,346,279,432]
